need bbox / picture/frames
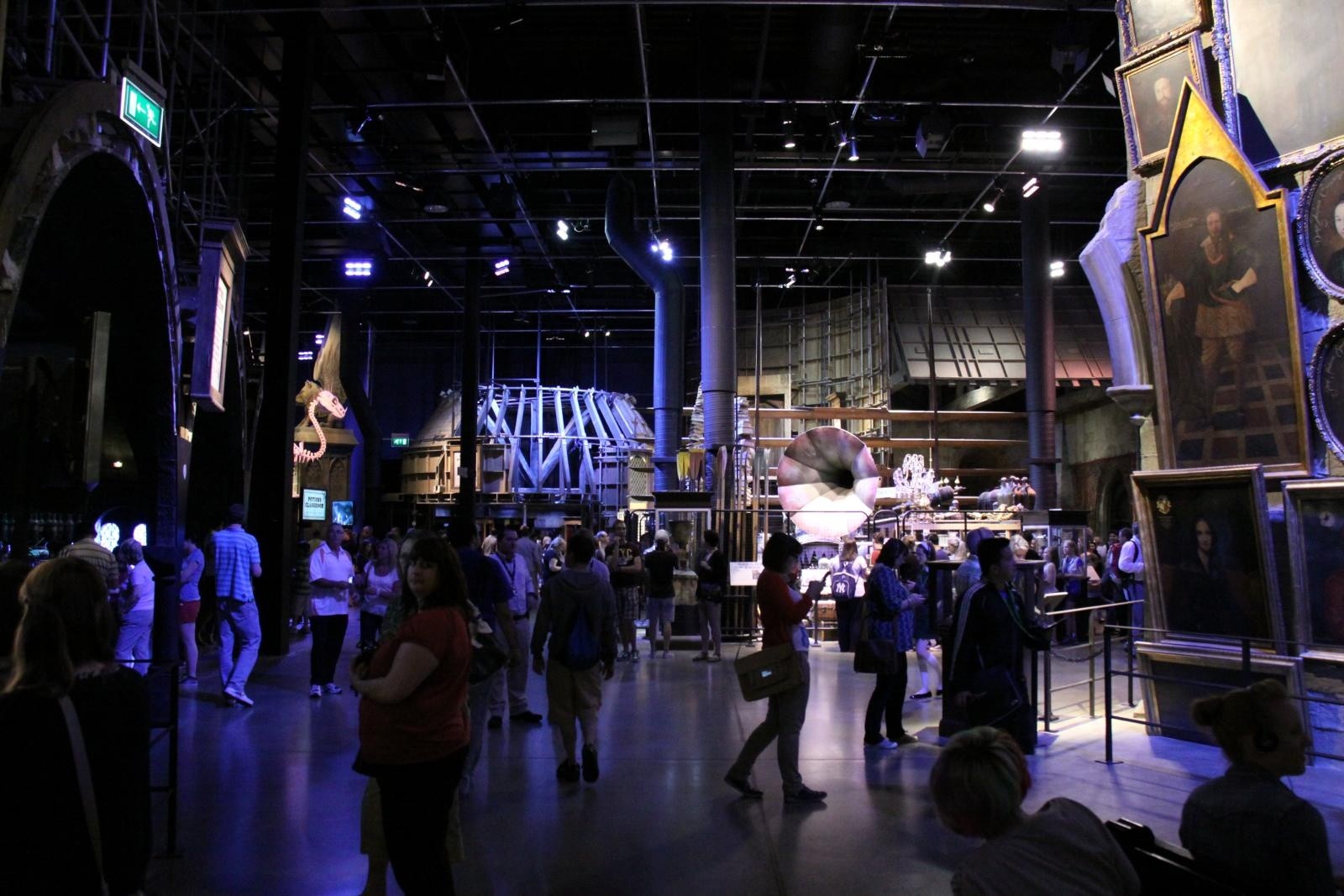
[1295,147,1343,306]
[1133,641,1316,768]
[1130,464,1289,657]
[1308,319,1344,465]
[1114,29,1217,173]
[1115,0,1209,61]
[1211,0,1344,179]
[1135,76,1313,478]
[1281,477,1344,662]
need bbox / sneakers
[783,783,827,802]
[556,759,580,784]
[323,683,342,693]
[631,650,638,662]
[864,738,898,748]
[647,652,658,658]
[662,651,674,659]
[616,651,630,662]
[706,654,720,662]
[724,770,764,798]
[487,715,502,730]
[691,652,708,661]
[892,732,918,743]
[308,684,322,697]
[508,711,542,724]
[179,676,198,686]
[581,743,599,783]
[224,685,254,706]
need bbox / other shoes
[909,691,932,699]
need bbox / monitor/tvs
[299,486,328,523]
[330,500,356,529]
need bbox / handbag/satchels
[732,641,801,701]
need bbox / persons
[1326,197,1344,291]
[1145,76,1185,152]
[1,489,1344,895]
[1160,213,1262,423]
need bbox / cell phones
[821,571,831,582]
